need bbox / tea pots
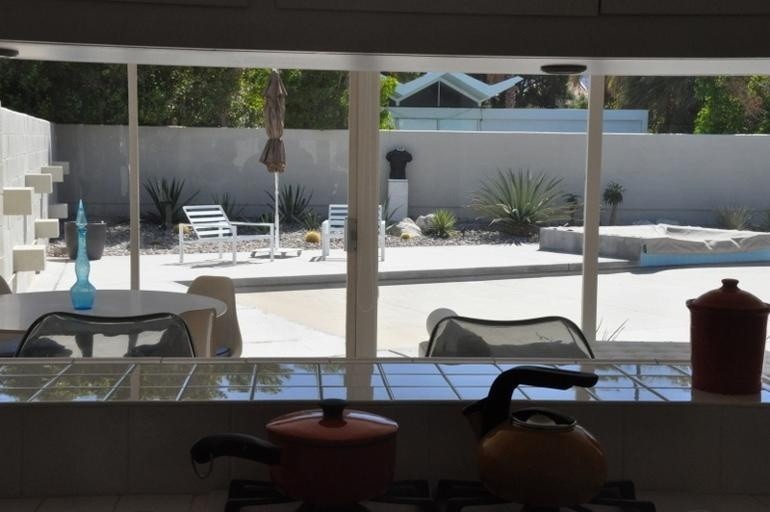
[462,366,609,507]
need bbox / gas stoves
[223,475,656,512]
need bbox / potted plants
[464,166,580,237]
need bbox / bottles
[70,198,96,310]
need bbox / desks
[0,289,229,357]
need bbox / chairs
[178,204,275,265]
[124,275,244,359]
[320,203,386,262]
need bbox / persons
[385,145,413,179]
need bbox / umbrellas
[256,68,290,249]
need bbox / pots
[190,399,401,505]
